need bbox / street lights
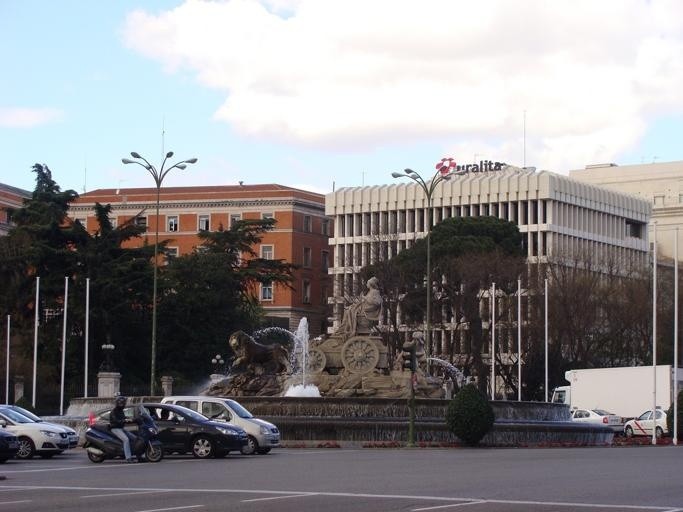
[212,353,225,374]
[120,148,197,395]
[391,166,469,375]
[97,342,118,373]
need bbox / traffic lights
[400,342,411,372]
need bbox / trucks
[547,363,682,418]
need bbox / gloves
[125,419,133,422]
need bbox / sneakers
[127,458,138,463]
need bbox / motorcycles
[82,405,164,465]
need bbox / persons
[339,277,380,332]
[110,398,148,463]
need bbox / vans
[155,394,281,457]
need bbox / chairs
[150,411,159,420]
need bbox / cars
[0,403,80,463]
[81,402,249,459]
[572,407,623,432]
[624,407,668,437]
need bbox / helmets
[114,396,127,408]
